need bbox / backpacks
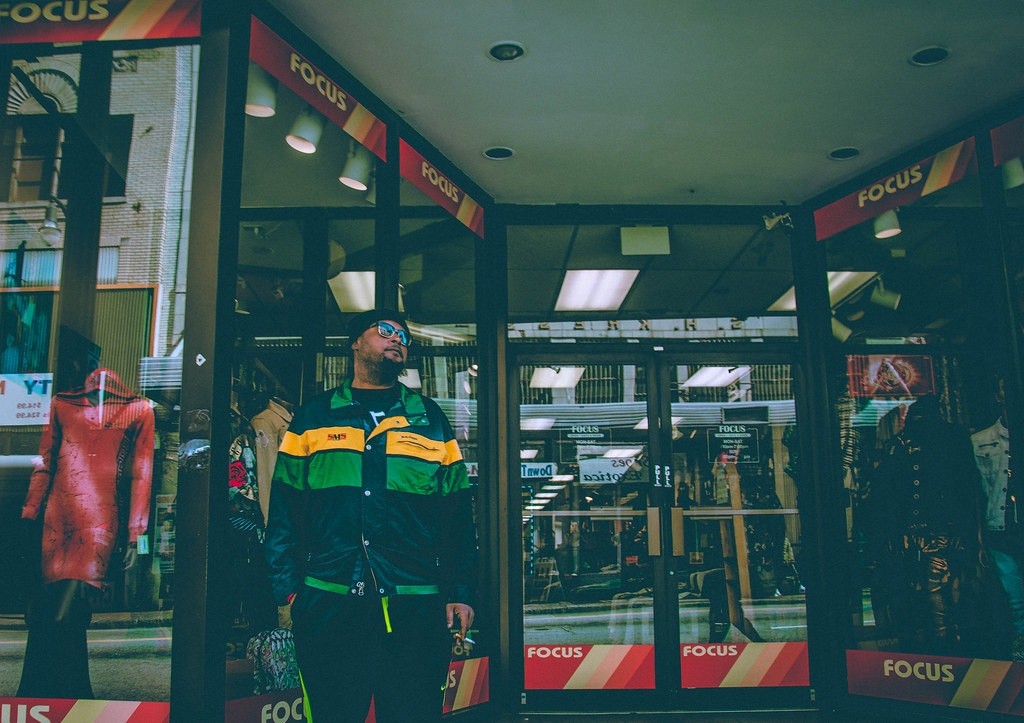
[246,623,301,696]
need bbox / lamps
[1001,155,1024,191]
[285,105,326,154]
[870,276,901,312]
[338,145,373,191]
[873,209,901,239]
[245,59,277,117]
[831,315,853,343]
[548,364,561,374]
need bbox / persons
[267,308,478,723]
[879,395,980,657]
[16,368,155,698]
[227,398,298,635]
[965,374,1023,659]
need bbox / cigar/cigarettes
[455,633,476,644]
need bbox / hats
[348,309,410,347]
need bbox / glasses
[365,321,412,347]
